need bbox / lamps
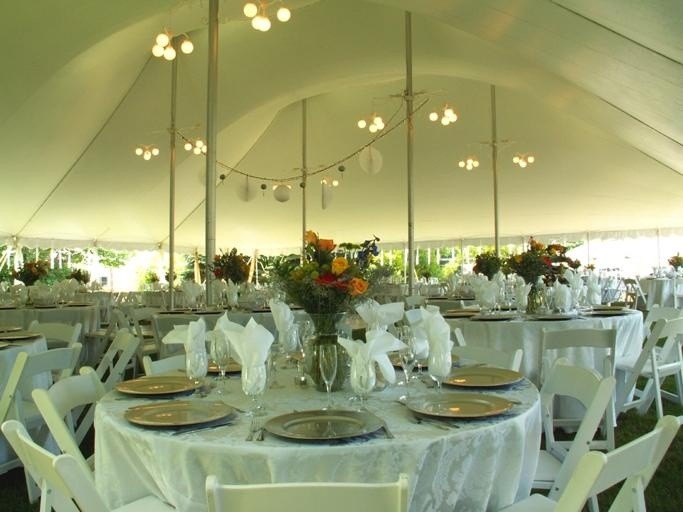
[427,92,457,127]
[183,137,207,158]
[510,143,533,172]
[242,1,291,32]
[273,182,293,197]
[457,154,479,173]
[356,103,385,136]
[322,174,339,186]
[152,27,195,62]
[136,142,161,162]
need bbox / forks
[245,419,261,441]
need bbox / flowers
[509,236,580,291]
[10,258,50,285]
[271,230,394,313]
[668,256,683,271]
[213,249,249,283]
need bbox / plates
[115,375,207,396]
[264,410,384,441]
[405,393,513,420]
[0,326,42,349]
[442,305,632,321]
[125,400,232,427]
[0,301,92,309]
[159,306,304,314]
[442,366,525,388]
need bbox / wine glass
[469,273,607,315]
[0,280,81,304]
[163,290,278,313]
[177,319,453,418]
